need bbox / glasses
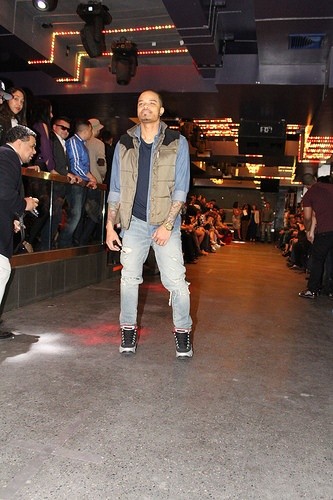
[0,95,6,101]
[57,124,71,132]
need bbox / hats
[89,119,104,131]
[0,80,14,101]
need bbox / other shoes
[0,331,15,340]
[298,290,315,300]
[19,243,31,254]
[249,238,256,242]
[187,234,226,264]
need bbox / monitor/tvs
[238,119,286,156]
[259,178,280,193]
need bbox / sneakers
[172,328,194,360]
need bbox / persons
[0,125,40,337]
[106,89,195,359]
[298,163,333,303]
[276,204,316,279]
[0,78,123,255]
[181,194,230,263]
[231,201,273,244]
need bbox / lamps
[108,36,139,85]
[33,0,58,12]
[76,0,113,58]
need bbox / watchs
[161,221,174,232]
